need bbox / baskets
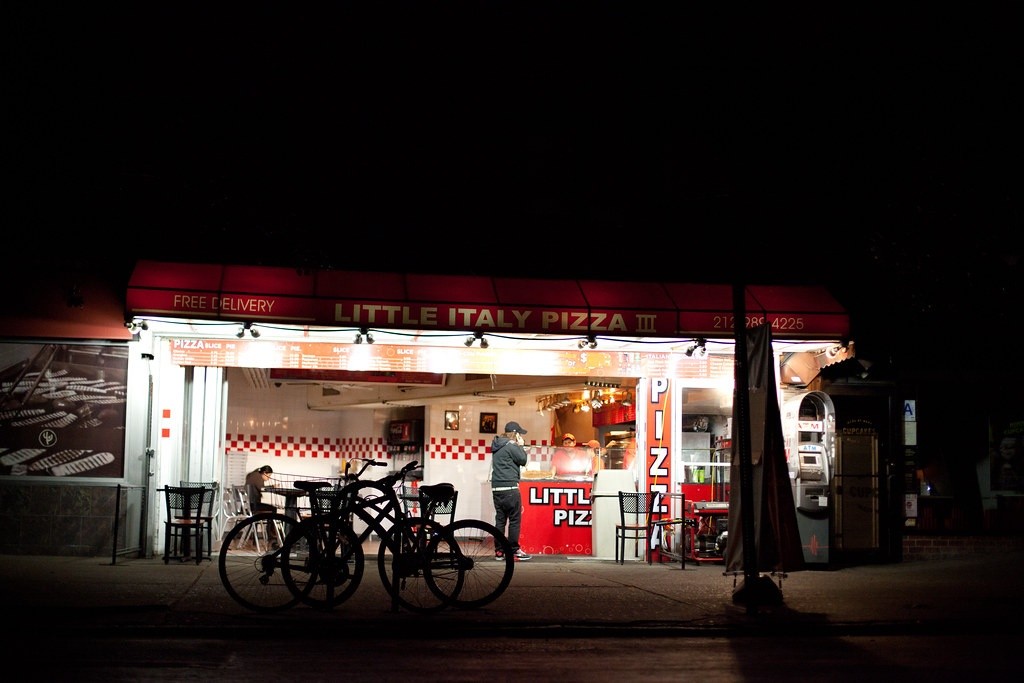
[311,484,351,511]
[263,469,341,514]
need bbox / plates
[693,501,729,510]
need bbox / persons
[240,465,281,549]
[548,432,606,477]
[490,421,533,561]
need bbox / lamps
[577,332,600,349]
[124,319,148,335]
[354,328,375,345]
[236,322,262,339]
[621,388,636,406]
[825,341,849,357]
[682,341,709,358]
[463,329,489,349]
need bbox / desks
[265,485,310,543]
[157,488,218,561]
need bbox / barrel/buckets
[692,465,706,483]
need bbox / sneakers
[494,549,506,561]
[504,548,532,561]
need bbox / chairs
[161,481,287,564]
[315,487,362,557]
[615,491,659,564]
[397,482,459,549]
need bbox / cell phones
[516,433,520,440]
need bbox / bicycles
[218,459,514,615]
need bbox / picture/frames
[479,412,498,433]
[443,410,459,431]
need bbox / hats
[505,421,528,434]
[582,439,600,448]
[561,433,575,441]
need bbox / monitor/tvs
[387,419,424,445]
